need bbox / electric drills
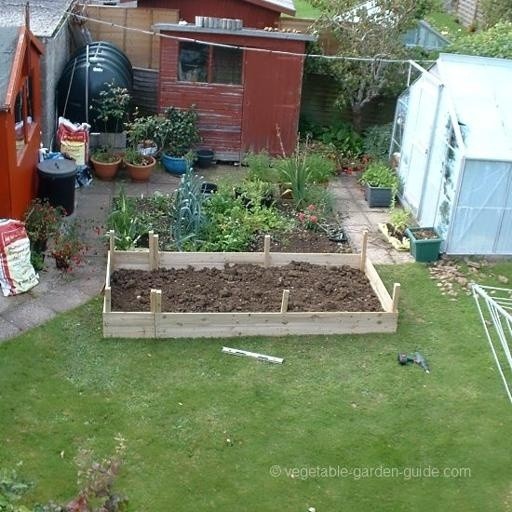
[400,351,430,375]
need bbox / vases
[202,182,218,194]
[404,226,443,263]
[197,150,214,167]
[25,224,49,269]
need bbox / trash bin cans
[35,159,78,217]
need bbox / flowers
[20,194,105,275]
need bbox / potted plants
[89,77,130,180]
[234,147,273,215]
[158,103,201,176]
[122,116,157,183]
[357,160,399,215]
